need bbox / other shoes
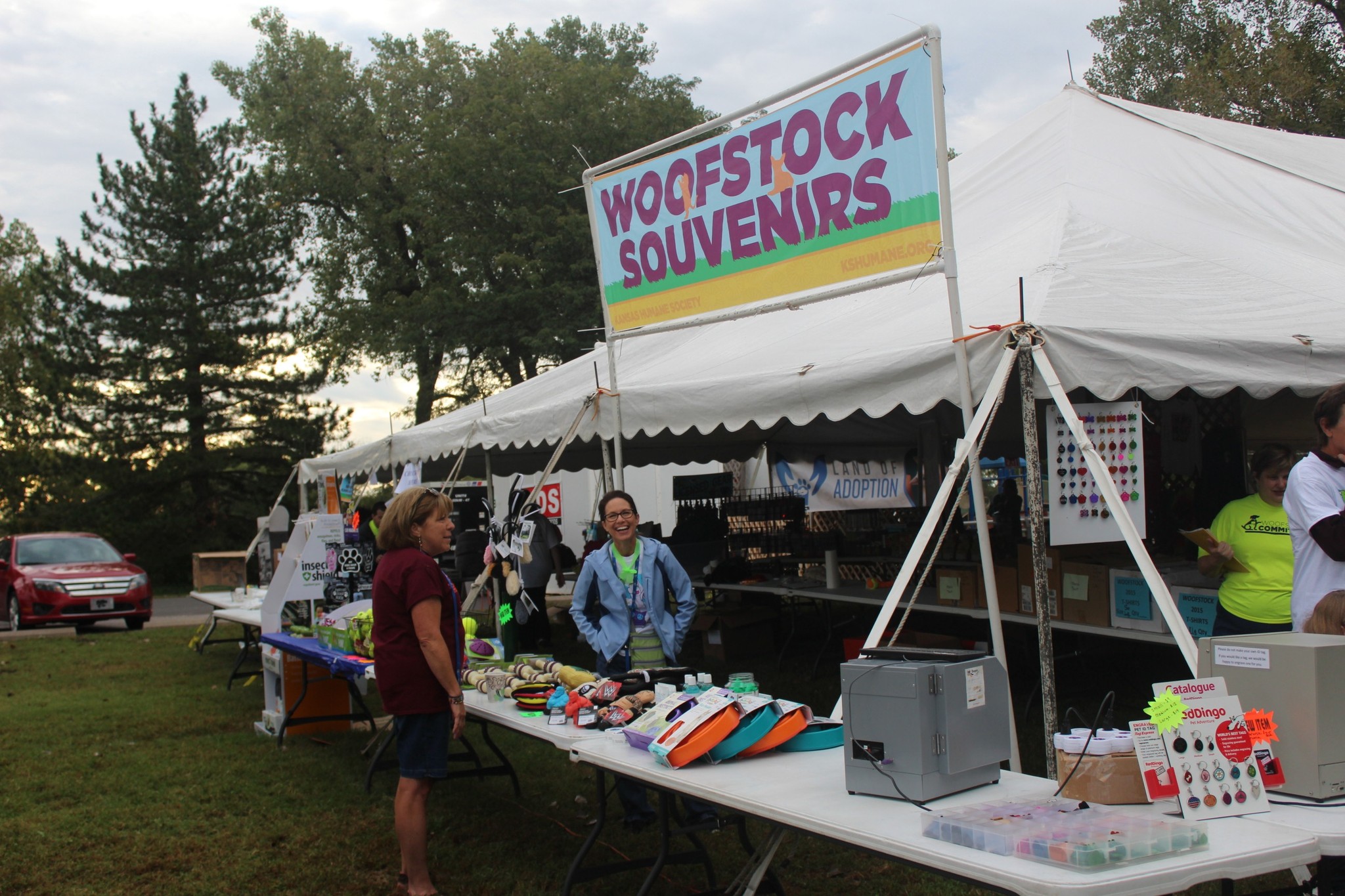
[396,871,448,895]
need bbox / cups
[236,587,244,601]
[247,585,258,601]
[484,672,507,703]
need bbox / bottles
[683,672,714,696]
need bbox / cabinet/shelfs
[252,514,354,742]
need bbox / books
[1178,527,1250,573]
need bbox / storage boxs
[843,627,983,662]
[318,623,356,655]
[1018,538,1129,621]
[1061,552,1178,627]
[977,563,1018,614]
[937,565,978,610]
[689,605,776,667]
[1109,559,1219,635]
[1171,581,1223,639]
[193,551,246,592]
[1055,747,1157,803]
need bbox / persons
[990,478,1025,561]
[1303,588,1344,635]
[366,487,468,896]
[1194,444,1294,637]
[1145,454,1178,558]
[362,501,387,584]
[502,488,569,651]
[1283,381,1345,635]
[571,488,701,830]
[453,484,496,596]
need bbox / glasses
[603,508,636,523]
[415,488,440,506]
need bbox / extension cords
[1052,725,1134,759]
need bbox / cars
[1,532,152,635]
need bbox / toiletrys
[687,676,700,696]
[682,673,695,693]
[696,672,707,689]
[701,673,715,693]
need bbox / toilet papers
[823,548,842,590]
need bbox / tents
[301,84,1343,780]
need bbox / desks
[754,555,937,625]
[363,669,717,894]
[578,724,1324,896]
[992,613,1227,728]
[212,609,264,690]
[259,632,488,788]
[692,574,995,685]
[188,589,267,656]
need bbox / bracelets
[448,693,464,706]
